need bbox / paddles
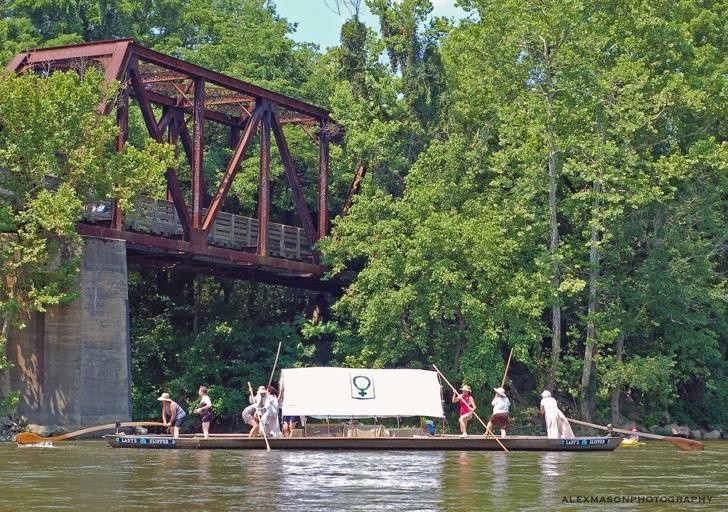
[16,422,171,444]
[565,418,704,452]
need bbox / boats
[102,430,624,450]
[620,439,646,446]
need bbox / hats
[460,385,472,393]
[541,391,552,397]
[257,386,267,394]
[158,393,172,402]
[493,387,506,396]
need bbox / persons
[157,393,186,439]
[483,387,510,436]
[242,385,307,439]
[193,386,215,438]
[452,385,476,437]
[629,425,640,442]
[540,390,576,439]
